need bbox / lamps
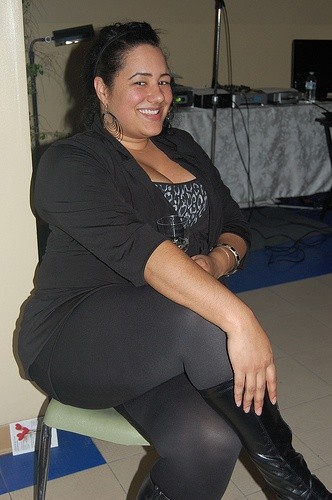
[30,24,96,146]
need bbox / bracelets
[207,241,241,279]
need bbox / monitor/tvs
[291,39,332,101]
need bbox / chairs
[33,396,152,499]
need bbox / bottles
[305,72,316,103]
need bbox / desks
[168,100,331,203]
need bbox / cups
[157,215,189,254]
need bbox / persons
[18,21,332,500]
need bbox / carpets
[218,190,332,293]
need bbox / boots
[136,472,170,500]
[197,377,332,500]
